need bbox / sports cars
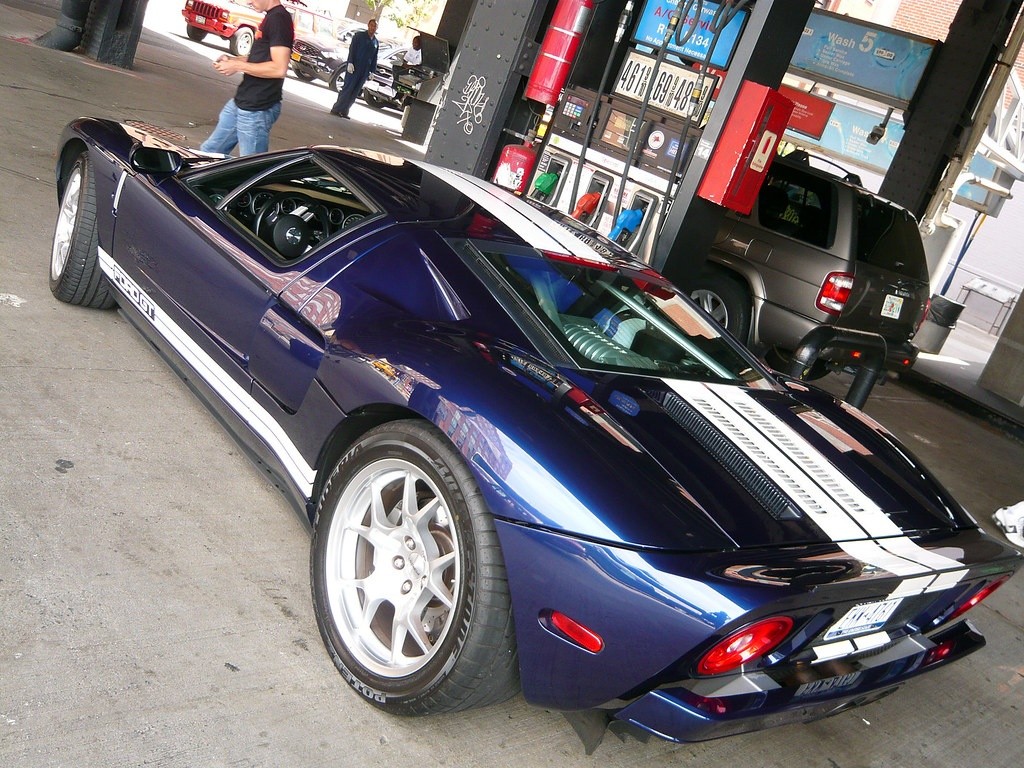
[51,115,1024,742]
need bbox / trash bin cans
[402,95,443,147]
[910,293,966,356]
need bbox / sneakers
[991,506,1024,548]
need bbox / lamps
[866,107,893,145]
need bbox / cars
[364,44,424,112]
[293,24,402,92]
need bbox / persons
[197,0,295,155]
[390,36,422,106]
[330,19,379,120]
[991,501,1024,547]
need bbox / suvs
[658,149,932,407]
[180,0,336,57]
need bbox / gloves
[347,63,355,74]
[366,73,374,82]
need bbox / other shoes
[341,113,350,120]
[392,92,404,100]
[330,110,343,118]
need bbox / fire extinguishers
[462,129,536,237]
[523,0,605,107]
